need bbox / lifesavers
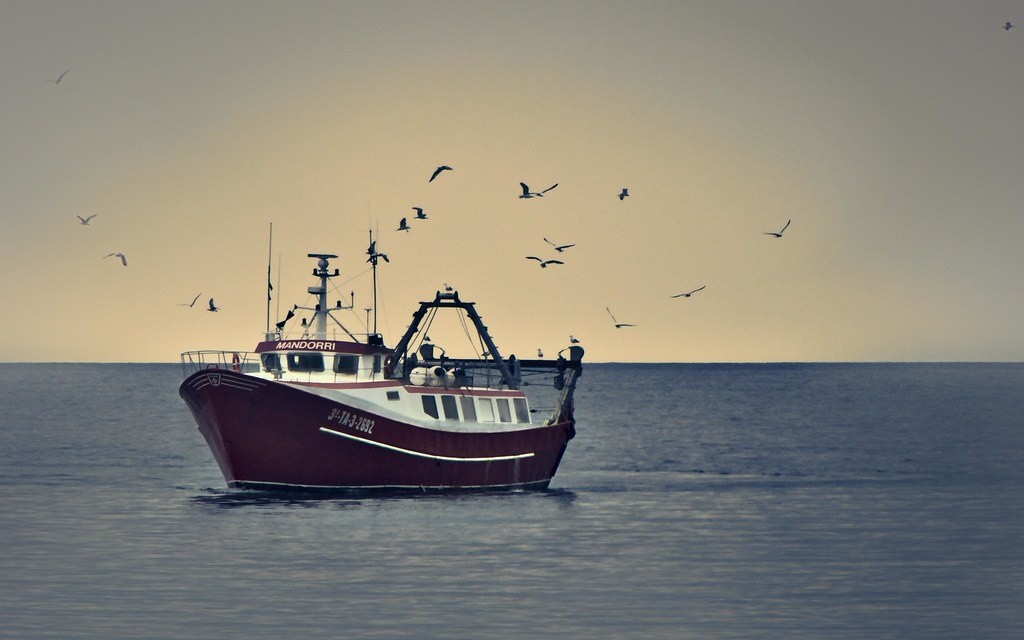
[232,353,240,373]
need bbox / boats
[180,221,588,499]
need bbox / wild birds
[396,218,411,232]
[525,238,575,268]
[206,298,220,312]
[519,182,558,199]
[177,293,202,307]
[617,188,629,200]
[570,335,580,344]
[101,252,127,266]
[607,307,638,328]
[762,219,791,237]
[77,215,96,225]
[429,166,453,182]
[1003,22,1015,31]
[412,207,428,219]
[538,349,543,357]
[670,286,706,298]
